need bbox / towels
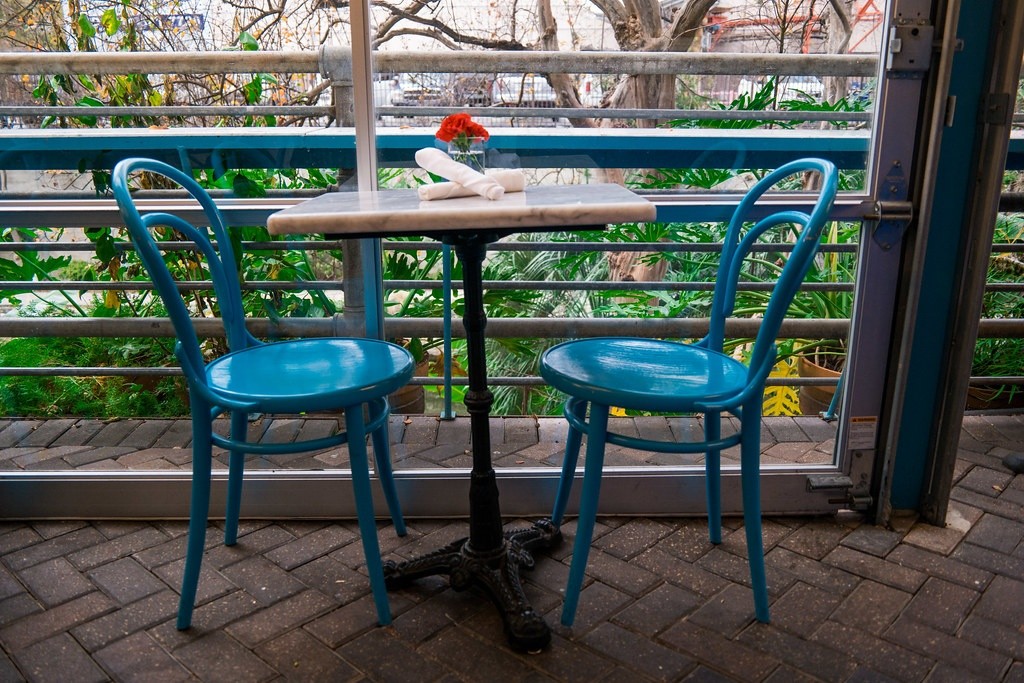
[416,168,526,202]
[413,147,506,202]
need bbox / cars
[493,71,602,123]
[389,73,447,118]
[738,71,824,110]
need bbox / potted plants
[744,189,861,411]
[383,238,464,418]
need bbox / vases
[447,138,487,174]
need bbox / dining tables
[265,178,658,660]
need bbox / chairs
[535,155,841,634]
[101,154,416,634]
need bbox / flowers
[435,113,490,173]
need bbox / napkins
[418,167,525,201]
[413,146,506,201]
